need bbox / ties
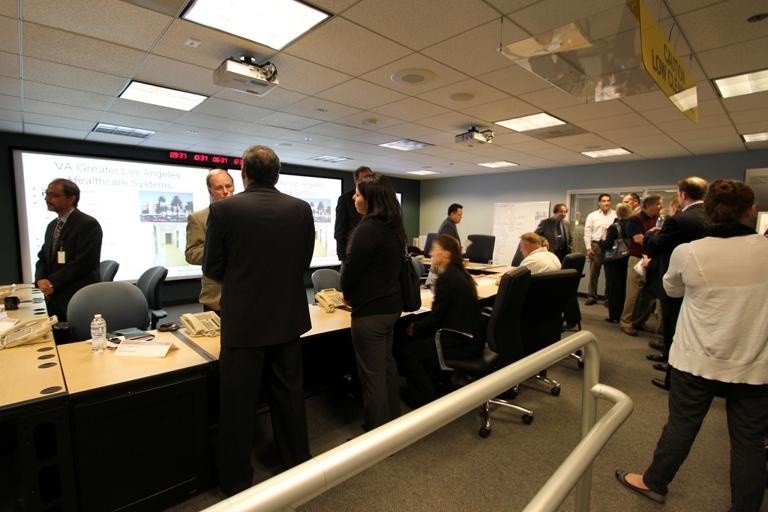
[51,219,63,263]
[557,221,563,250]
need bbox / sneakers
[615,469,666,504]
[584,297,596,305]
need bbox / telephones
[180,311,220,337]
[316,287,346,312]
[0,314,58,351]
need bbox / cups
[3,296,19,310]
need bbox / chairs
[311,269,342,303]
[67,281,149,341]
[561,252,586,369]
[430,266,534,438]
[136,266,168,330]
[406,245,432,284]
[100,260,119,282]
[460,234,496,275]
[511,268,579,399]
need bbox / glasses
[42,192,65,199]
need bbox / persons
[534,204,575,265]
[35,178,102,345]
[334,166,374,263]
[400,234,479,410]
[201,146,315,501]
[614,179,768,512]
[520,232,562,275]
[185,169,234,317]
[439,203,463,251]
[339,178,408,433]
[583,176,710,391]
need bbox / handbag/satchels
[402,254,420,311]
[604,239,630,262]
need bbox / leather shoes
[648,340,666,390]
[620,327,638,336]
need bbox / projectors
[212,58,279,96]
[455,126,494,149]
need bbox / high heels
[606,317,620,323]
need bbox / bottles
[89,313,107,353]
[656,215,664,228]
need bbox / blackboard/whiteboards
[492,201,550,267]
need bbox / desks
[56,260,520,512]
[0,282,69,512]
